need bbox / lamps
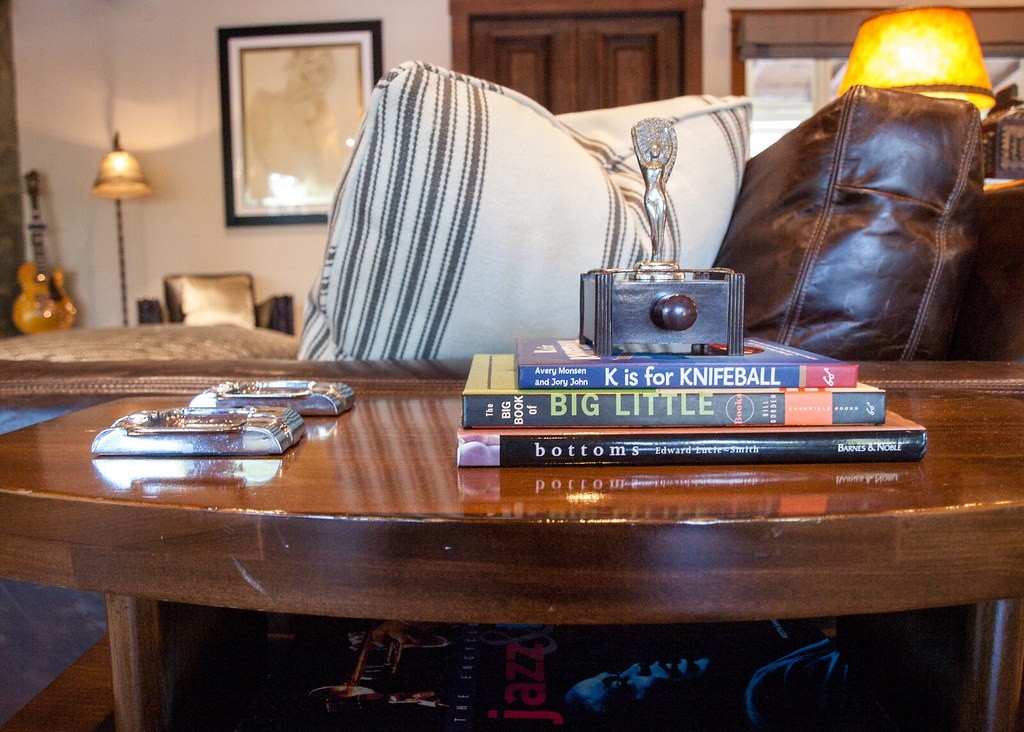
[88,132,152,326]
[838,5,996,115]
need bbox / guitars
[7,169,78,336]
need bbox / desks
[0,394,1024,732]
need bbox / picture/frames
[217,19,384,228]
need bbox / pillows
[948,178,1023,363]
[706,86,985,361]
[298,58,756,361]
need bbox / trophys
[630,116,685,280]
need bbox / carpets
[1,407,110,724]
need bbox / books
[456,336,928,468]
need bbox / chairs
[140,273,300,335]
